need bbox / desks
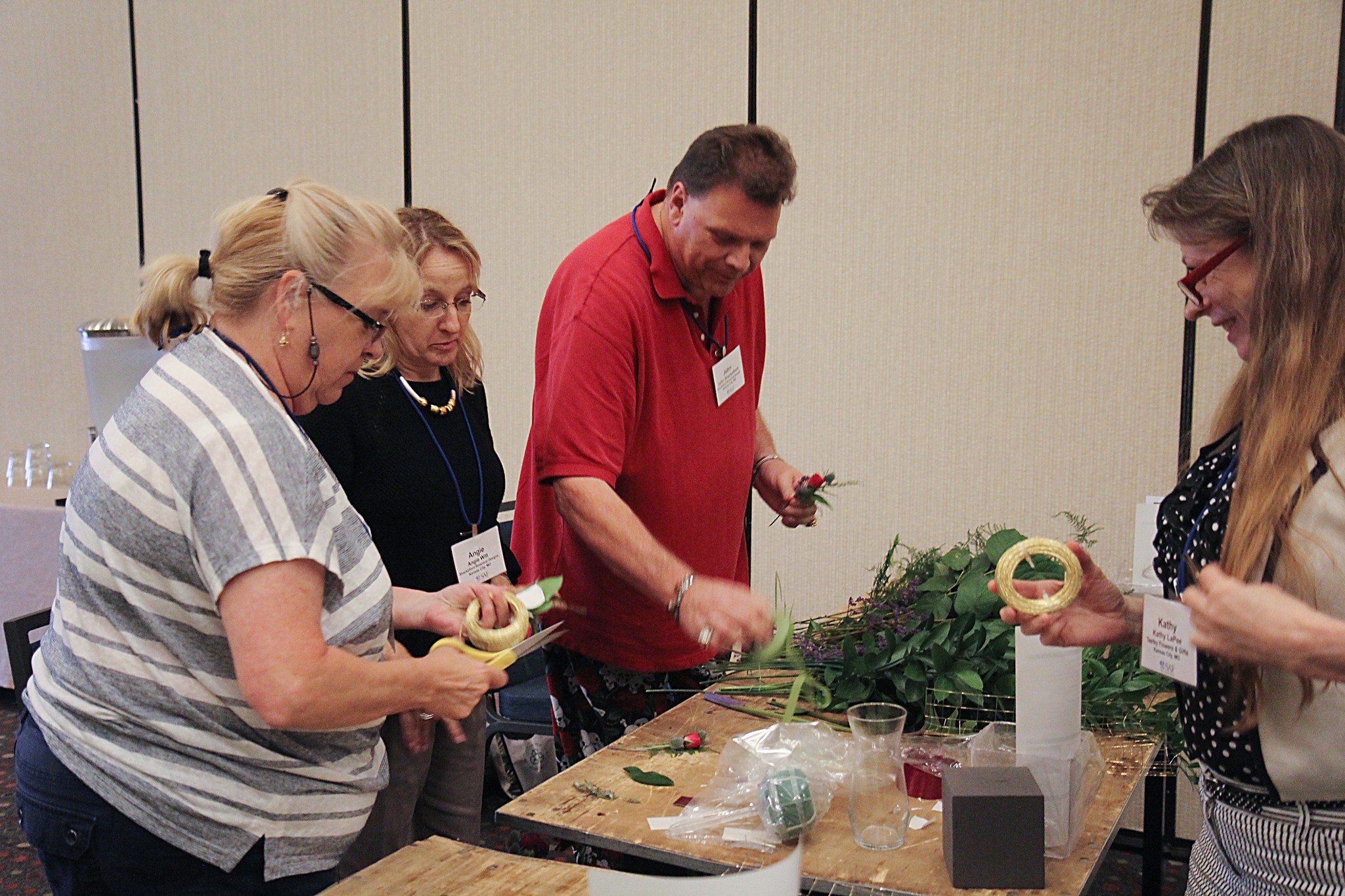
[316,834,606,896]
[494,612,1170,896]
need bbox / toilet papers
[1014,626,1084,847]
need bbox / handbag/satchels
[486,721,559,798]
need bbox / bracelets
[667,572,698,627]
[753,455,783,476]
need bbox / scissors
[429,620,568,711]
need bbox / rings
[421,712,433,720]
[805,517,817,528]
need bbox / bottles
[846,702,912,852]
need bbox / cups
[6,442,72,489]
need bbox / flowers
[633,728,708,756]
[701,571,925,682]
[769,470,858,536]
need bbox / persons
[290,208,521,851]
[510,124,819,871]
[987,116,1345,896]
[12,180,515,896]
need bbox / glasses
[414,287,488,319]
[1176,231,1250,309]
[310,281,389,344]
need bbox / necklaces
[212,328,374,538]
[395,367,456,415]
